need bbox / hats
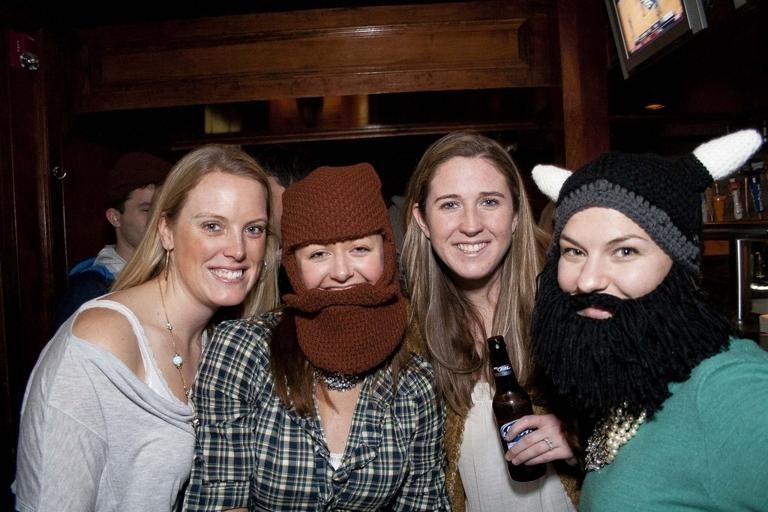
[95,150,171,220]
[282,164,395,252]
[531,129,762,282]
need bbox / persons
[180,162,453,511]
[58,151,172,325]
[8,141,283,512]
[395,129,581,511]
[525,126,768,512]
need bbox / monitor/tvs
[606,0,711,81]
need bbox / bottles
[485,334,549,487]
[703,173,768,224]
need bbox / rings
[544,438,554,450]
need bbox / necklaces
[318,369,359,391]
[157,276,190,399]
